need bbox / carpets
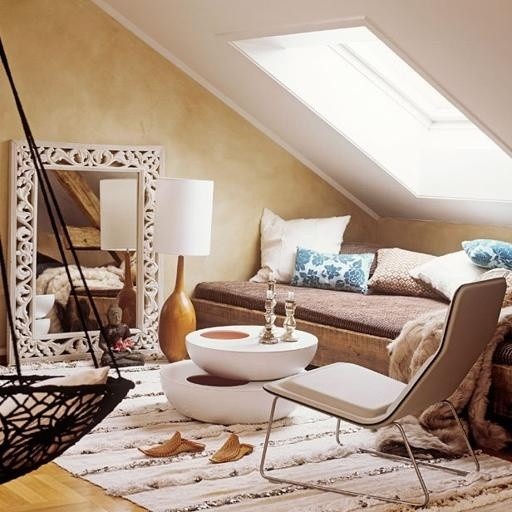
[0,363,512,512]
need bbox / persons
[99,304,143,366]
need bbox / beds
[189,210,511,435]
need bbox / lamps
[97,180,138,330]
[156,177,215,364]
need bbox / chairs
[258,276,509,507]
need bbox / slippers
[144,431,206,457]
[209,434,254,463]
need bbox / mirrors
[7,139,169,370]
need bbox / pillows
[248,208,512,305]
